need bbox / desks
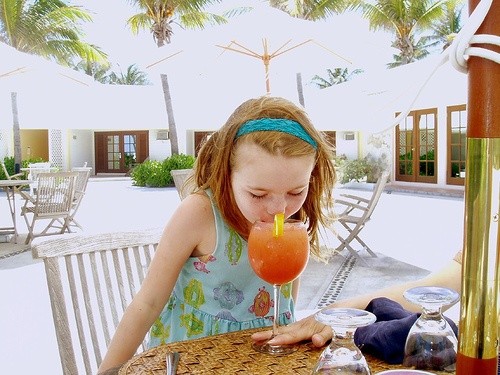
[101,322,457,375]
[0,180,34,245]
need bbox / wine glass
[309,307,377,375]
[247,217,310,355]
[400,285,459,375]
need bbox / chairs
[31,228,165,375]
[318,171,391,267]
[170,169,199,201]
[19,162,93,245]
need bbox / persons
[96,96,342,375]
[250,249,461,346]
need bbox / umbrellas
[143,7,355,95]
[0,41,95,92]
